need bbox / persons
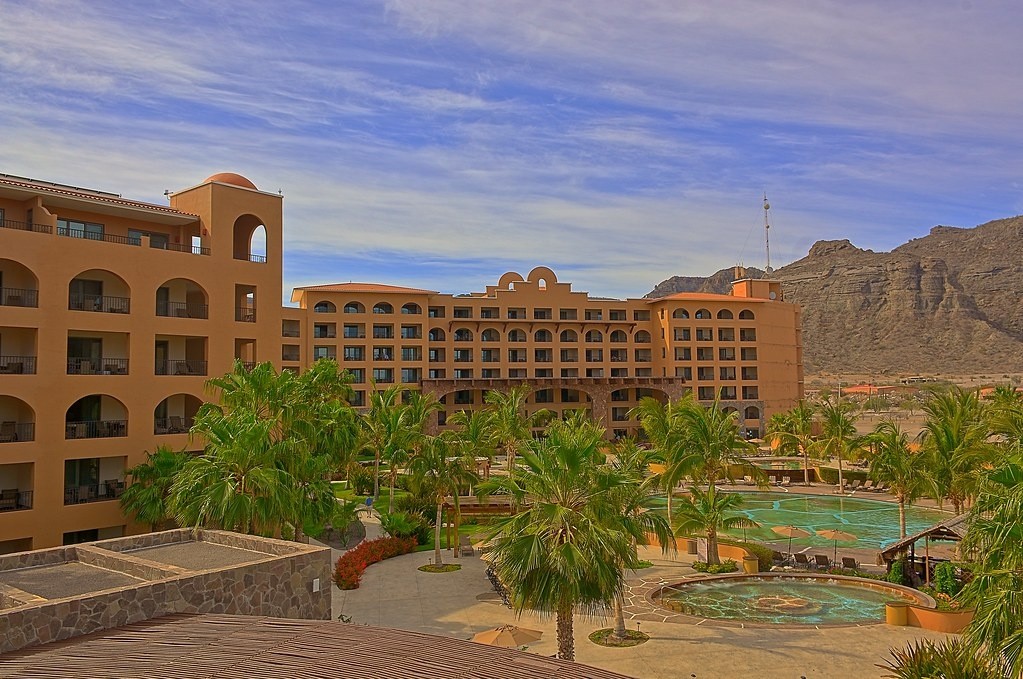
[364,495,373,518]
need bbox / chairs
[75,424,87,438]
[0,488,20,511]
[867,481,885,493]
[0,421,18,442]
[773,551,792,565]
[96,421,124,437]
[74,484,89,504]
[105,479,123,499]
[5,362,23,374]
[780,476,790,487]
[857,480,873,492]
[79,360,91,375]
[168,416,188,433]
[842,557,861,569]
[814,555,833,568]
[845,480,861,492]
[175,362,189,375]
[794,553,815,567]
[769,476,777,485]
[458,533,474,557]
[744,476,753,486]
[835,479,848,491]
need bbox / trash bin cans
[743,556,759,573]
[687,538,697,554]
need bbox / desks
[7,295,22,306]
[176,308,191,317]
[106,364,119,375]
[74,363,95,375]
[84,298,95,311]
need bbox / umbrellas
[771,525,811,567]
[817,529,858,567]
[728,519,763,543]
